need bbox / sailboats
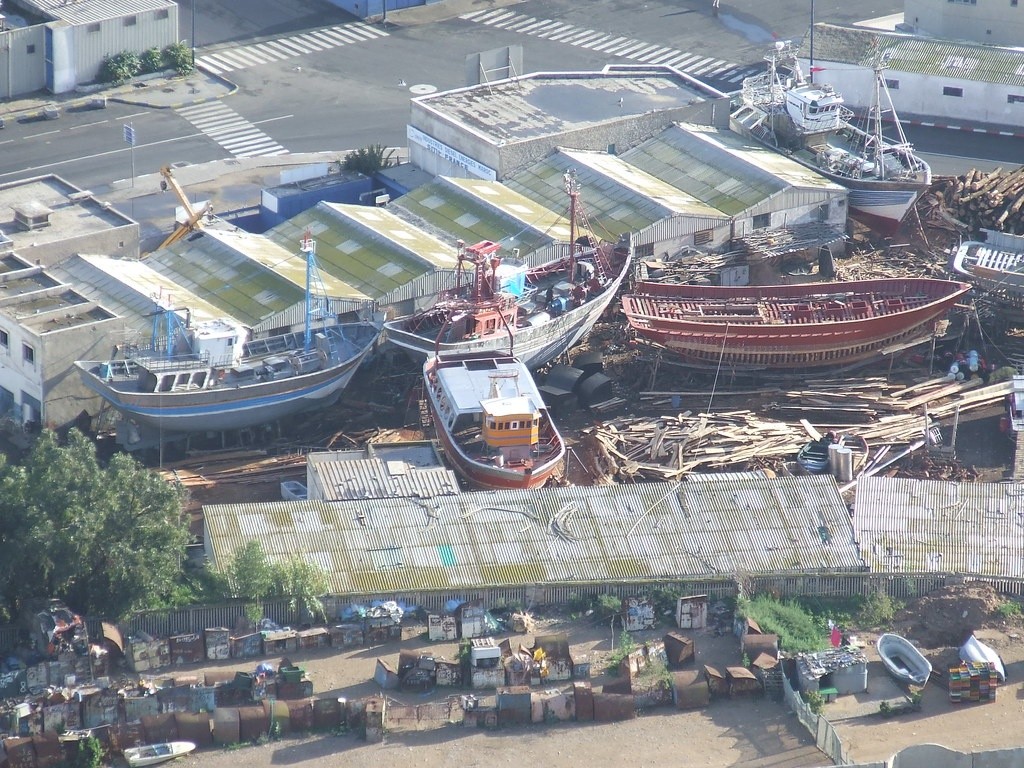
[726,0,934,237]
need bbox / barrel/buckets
[672,396,682,410]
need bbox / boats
[422,273,566,490]
[70,224,388,436]
[796,439,831,473]
[953,226,1024,324]
[876,633,932,689]
[958,635,1009,683]
[839,434,870,472]
[618,274,974,373]
[280,479,308,502]
[120,738,197,768]
[380,164,636,369]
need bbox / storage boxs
[0,593,783,768]
[945,660,999,703]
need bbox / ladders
[576,202,608,284]
[308,256,341,364]
[478,266,482,301]
[841,76,875,177]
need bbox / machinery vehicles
[139,164,217,258]
